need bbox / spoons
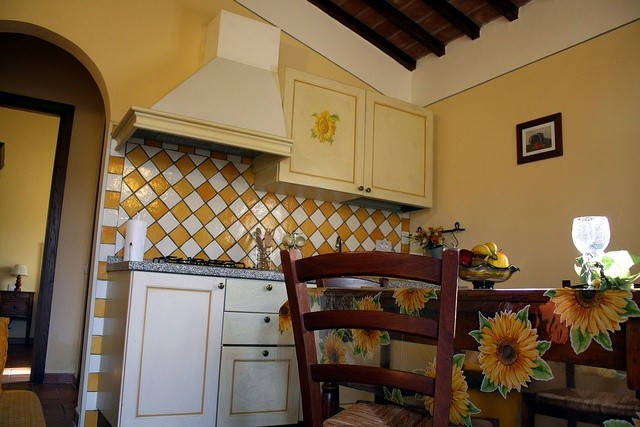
[255,227,262,236]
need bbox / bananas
[470,241,498,259]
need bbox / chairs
[280,246,459,427]
[317,274,391,410]
[518,280,640,427]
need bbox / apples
[459,249,473,266]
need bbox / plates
[458,260,520,291]
[321,274,385,291]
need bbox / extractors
[110,8,293,161]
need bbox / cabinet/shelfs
[219,277,301,427]
[118,268,227,426]
[282,68,433,208]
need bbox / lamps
[11,264,28,289]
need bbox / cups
[295,233,306,250]
[281,233,294,248]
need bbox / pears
[488,249,508,268]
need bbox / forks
[264,227,274,247]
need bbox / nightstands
[2,290,35,342]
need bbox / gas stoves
[153,254,249,277]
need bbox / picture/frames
[516,111,563,165]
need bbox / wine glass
[569,214,611,289]
